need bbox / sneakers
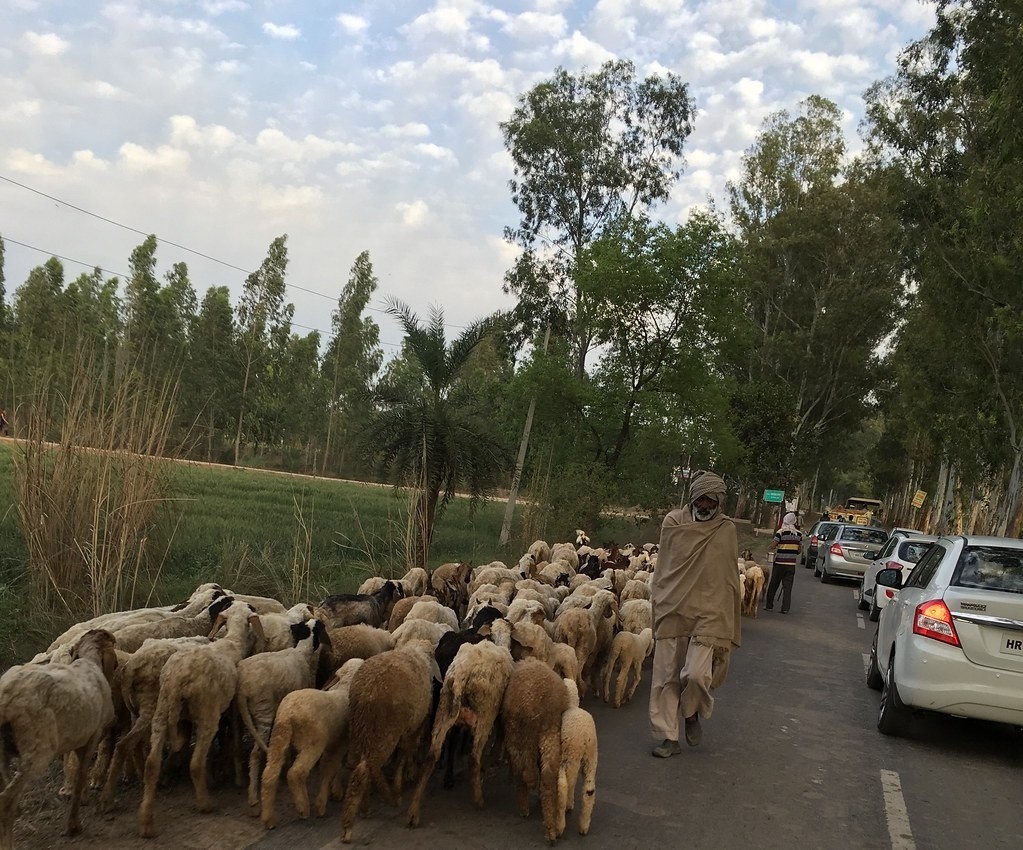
[685,711,703,747]
[652,739,681,758]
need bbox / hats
[823,511,829,515]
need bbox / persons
[649,470,741,758]
[762,513,802,614]
[846,515,855,524]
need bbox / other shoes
[768,604,773,612]
[781,606,788,614]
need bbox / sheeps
[0,540,771,850]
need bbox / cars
[889,527,924,538]
[814,525,890,584]
[800,521,850,569]
[867,535,1023,738]
[858,531,943,622]
